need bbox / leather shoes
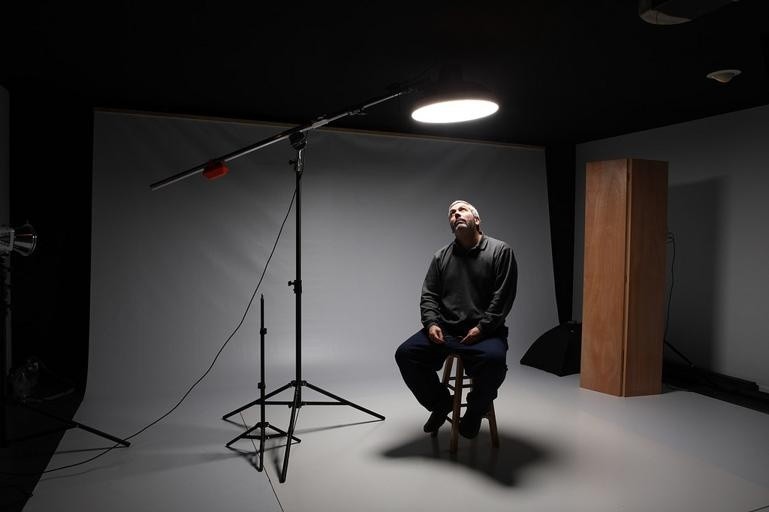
[460,408,482,438]
[424,397,453,432]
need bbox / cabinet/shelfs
[580,157,670,398]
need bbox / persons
[394,200,519,440]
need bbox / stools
[442,353,499,458]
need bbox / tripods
[149,89,414,483]
[1,257,130,447]
[224,293,301,472]
